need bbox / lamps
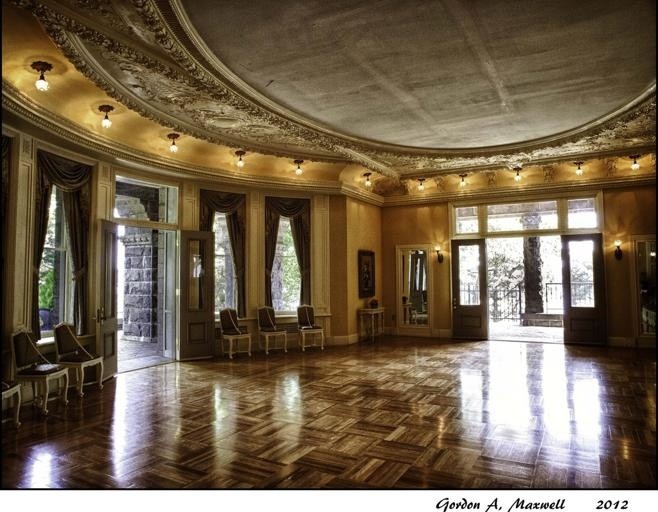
[434,244,443,264]
[513,168,522,182]
[294,159,304,176]
[459,174,467,187]
[235,151,246,168]
[629,154,641,170]
[573,162,584,176]
[418,179,425,192]
[167,133,180,153]
[98,105,114,129]
[31,61,54,91]
[614,241,623,261]
[363,173,372,187]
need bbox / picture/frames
[358,249,375,299]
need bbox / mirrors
[627,234,656,339]
[395,244,433,328]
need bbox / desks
[359,307,384,344]
[401,303,411,325]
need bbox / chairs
[219,307,253,359]
[0,377,22,429]
[54,321,105,397]
[11,322,71,415]
[296,304,324,352]
[256,305,288,355]
